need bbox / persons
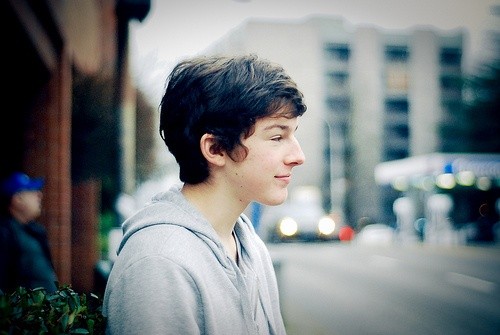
[0,173,58,292]
[102,56,307,335]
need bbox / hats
[2,173,44,196]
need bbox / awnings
[375,153,500,185]
